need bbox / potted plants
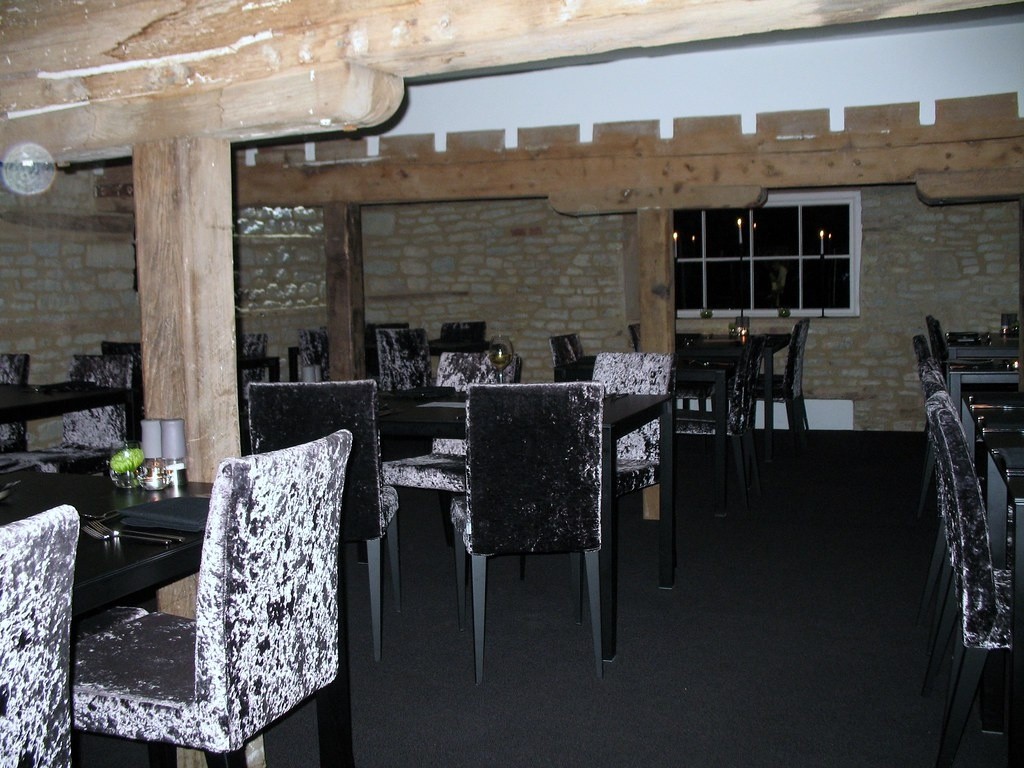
[110,448,144,488]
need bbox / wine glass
[488,335,514,384]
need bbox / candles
[159,418,186,460]
[141,419,162,458]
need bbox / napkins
[117,497,210,533]
[399,385,456,402]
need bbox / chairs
[0,318,809,768]
[912,315,1008,701]
[925,390,1024,768]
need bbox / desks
[553,355,730,518]
[0,380,135,445]
[0,469,214,617]
[377,384,677,662]
[945,331,1024,767]
[365,339,487,354]
[675,333,792,472]
[237,356,283,411]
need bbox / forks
[80,520,185,545]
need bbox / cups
[301,364,322,382]
[729,321,737,336]
[109,419,189,491]
[700,308,712,319]
[779,308,790,318]
